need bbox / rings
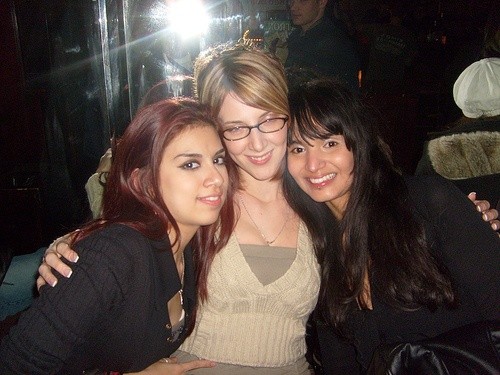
[163,357,169,364]
[43,251,58,263]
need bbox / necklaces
[168,229,184,304]
[238,193,289,247]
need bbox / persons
[277,0,382,117]
[0,95,245,375]
[416,52,500,233]
[282,76,500,375]
[36,40,500,375]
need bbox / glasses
[217,116,289,141]
